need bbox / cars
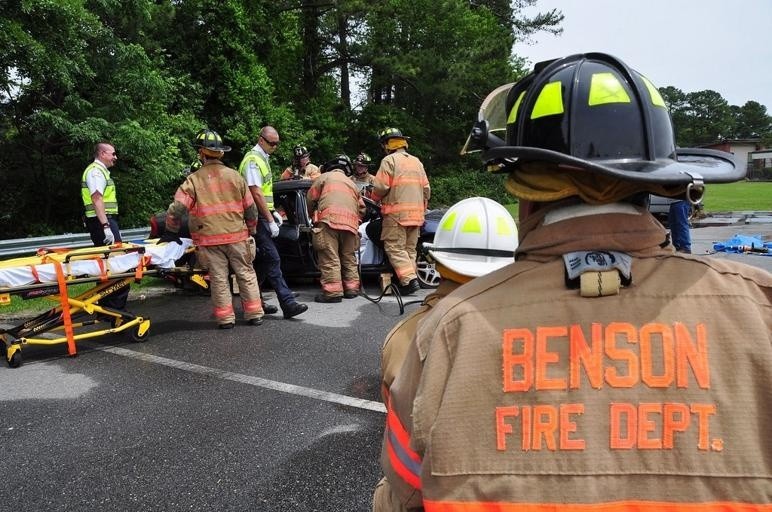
[648,194,705,220]
[149,181,449,289]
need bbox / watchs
[102,222,112,227]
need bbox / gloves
[269,220,278,238]
[273,211,283,227]
[155,229,182,245]
[103,227,115,245]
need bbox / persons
[305,153,366,304]
[163,127,266,331]
[79,143,131,321]
[368,124,432,296]
[237,126,310,319]
[347,152,375,183]
[371,196,520,511]
[378,48,771,512]
[667,198,693,256]
[281,143,321,180]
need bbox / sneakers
[262,303,278,314]
[315,293,342,303]
[284,302,308,320]
[250,318,263,325]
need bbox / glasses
[261,135,279,146]
[107,151,116,156]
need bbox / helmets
[461,52,748,188]
[193,129,231,152]
[375,127,411,143]
[422,197,520,277]
[354,152,376,166]
[320,154,353,177]
[295,146,312,158]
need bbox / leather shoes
[401,279,420,295]
